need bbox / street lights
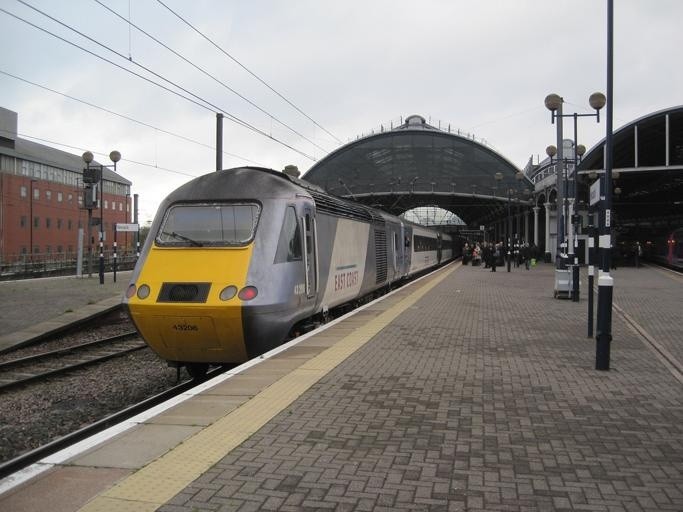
[123,193,130,254]
[540,91,606,301]
[81,149,121,284]
[28,177,37,262]
[494,171,525,273]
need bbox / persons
[461,240,541,273]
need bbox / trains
[122,163,465,378]
[620,227,682,271]
